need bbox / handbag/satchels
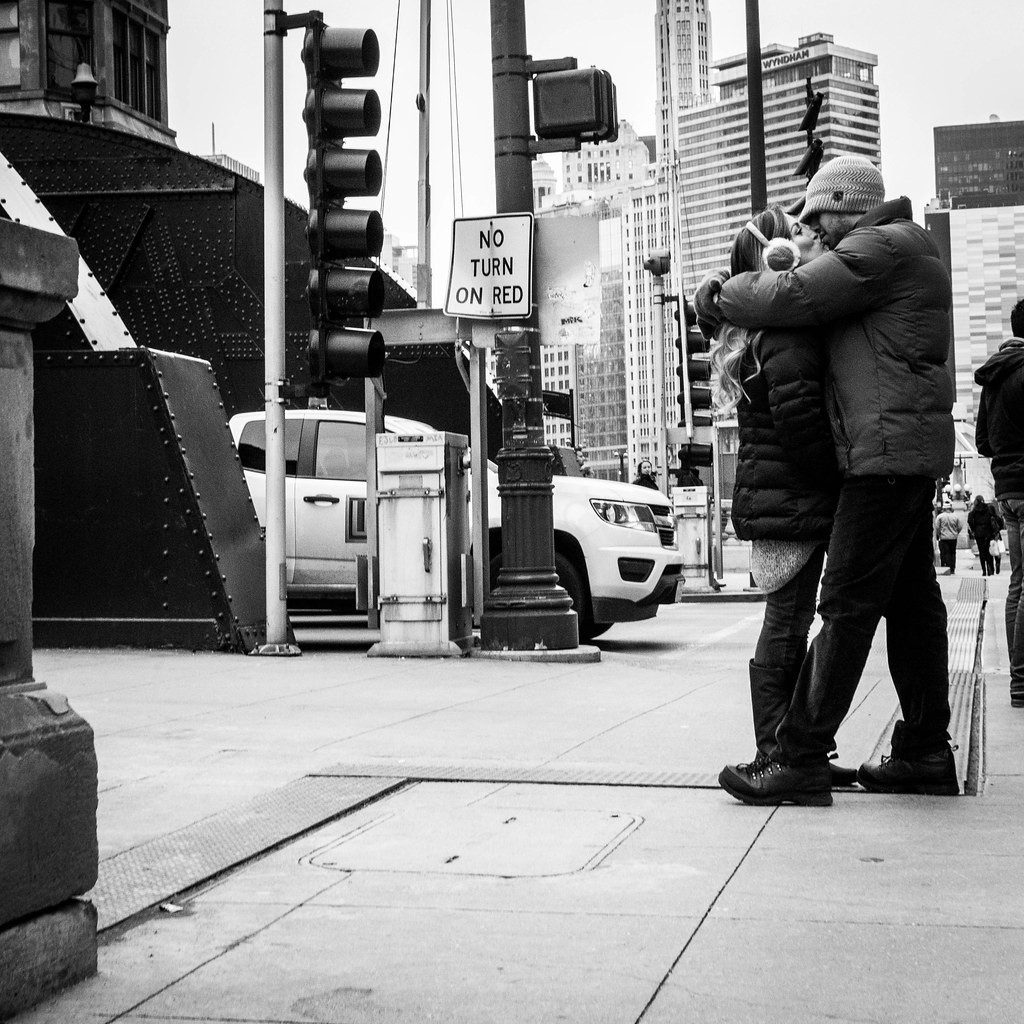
[989,539,1005,557]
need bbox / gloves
[693,269,730,327]
[695,315,720,340]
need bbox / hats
[797,155,885,224]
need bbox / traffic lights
[675,304,712,467]
[303,25,385,385]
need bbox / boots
[748,658,801,762]
[827,752,857,785]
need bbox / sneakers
[856,745,960,796]
[718,757,832,806]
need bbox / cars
[228,410,683,645]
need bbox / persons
[730,207,857,787]
[632,461,659,490]
[574,448,593,478]
[695,157,959,805]
[967,496,996,576]
[936,501,962,574]
[989,506,1003,575]
[974,299,1024,707]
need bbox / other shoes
[1010,693,1024,707]
